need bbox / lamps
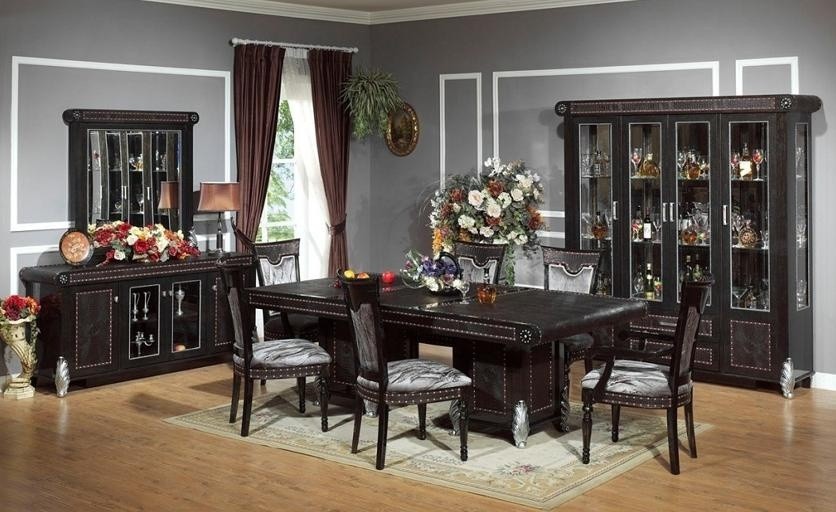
[197,182,241,254]
[157,181,179,232]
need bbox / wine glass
[582,145,808,308]
[112,150,167,212]
[459,276,470,303]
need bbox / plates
[59,227,95,266]
[335,268,380,286]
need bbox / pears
[344,270,355,278]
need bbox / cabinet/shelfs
[87,128,179,232]
[20,249,254,388]
[555,94,822,399]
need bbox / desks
[246,271,650,448]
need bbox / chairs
[453,240,508,284]
[336,269,474,470]
[250,239,322,385]
[216,262,332,437]
[580,279,713,475]
[540,245,605,377]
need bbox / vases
[424,275,448,293]
[0,316,36,399]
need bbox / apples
[357,272,370,279]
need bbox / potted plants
[334,65,402,144]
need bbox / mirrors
[64,108,200,260]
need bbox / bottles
[478,269,496,305]
[592,142,755,309]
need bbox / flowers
[399,260,454,282]
[415,158,546,259]
[92,220,200,267]
[0,294,42,321]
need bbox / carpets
[163,378,713,511]
